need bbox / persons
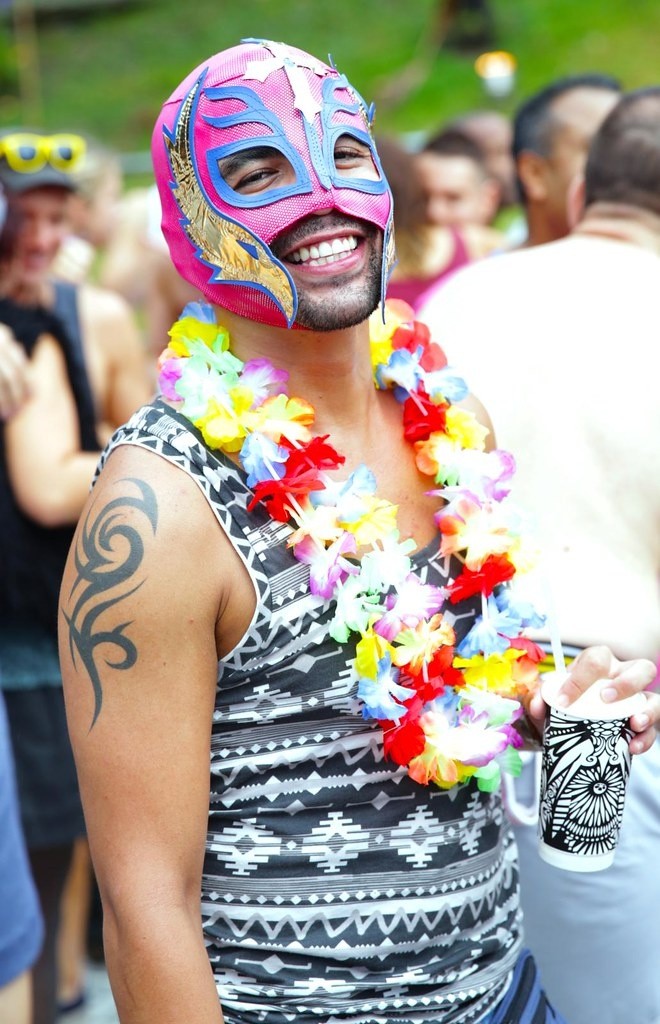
[0,38,660,1024]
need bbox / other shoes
[55,989,84,1014]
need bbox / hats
[0,128,81,193]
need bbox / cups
[539,670,648,872]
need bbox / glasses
[0,132,87,174]
